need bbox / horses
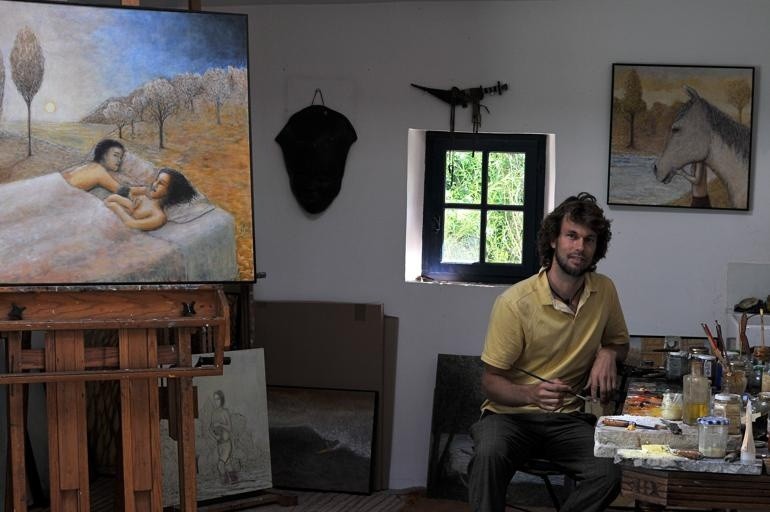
[650,83,750,210]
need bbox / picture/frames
[266,384,379,497]
[606,63,756,211]
[0,2,257,286]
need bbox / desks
[616,377,770,512]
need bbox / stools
[506,461,575,512]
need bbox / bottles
[662,346,770,459]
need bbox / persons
[207,392,241,489]
[672,160,710,208]
[61,138,197,231]
[462,194,623,512]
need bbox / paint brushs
[506,362,588,405]
[699,321,730,364]
[758,308,766,349]
